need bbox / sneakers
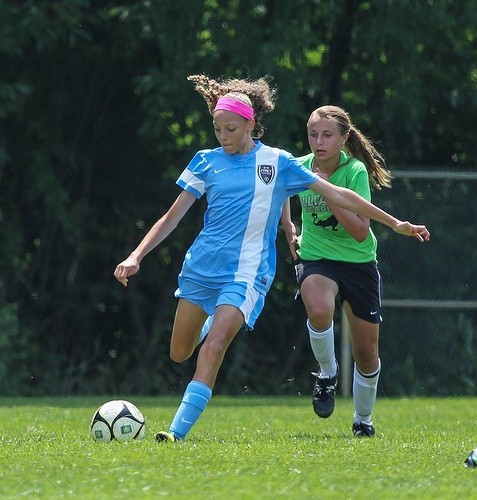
[351,420,375,438]
[312,358,339,418]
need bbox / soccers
[90,399,145,443]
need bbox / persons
[283,106,394,438]
[113,74,431,443]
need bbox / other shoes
[155,431,176,444]
